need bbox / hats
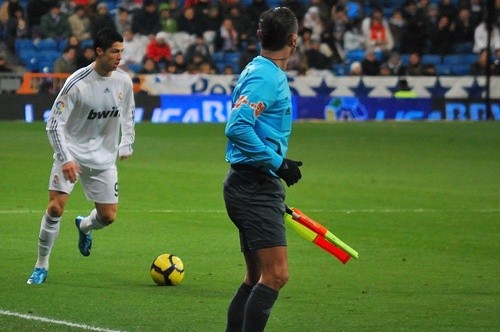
[350,62,360,70]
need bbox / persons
[26,28,136,285]
[0,0,268,76]
[223,7,303,332]
[275,0,500,76]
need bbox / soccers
[150,254,184,286]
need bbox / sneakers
[75,216,92,256]
[27,267,47,284]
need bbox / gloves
[274,158,302,187]
